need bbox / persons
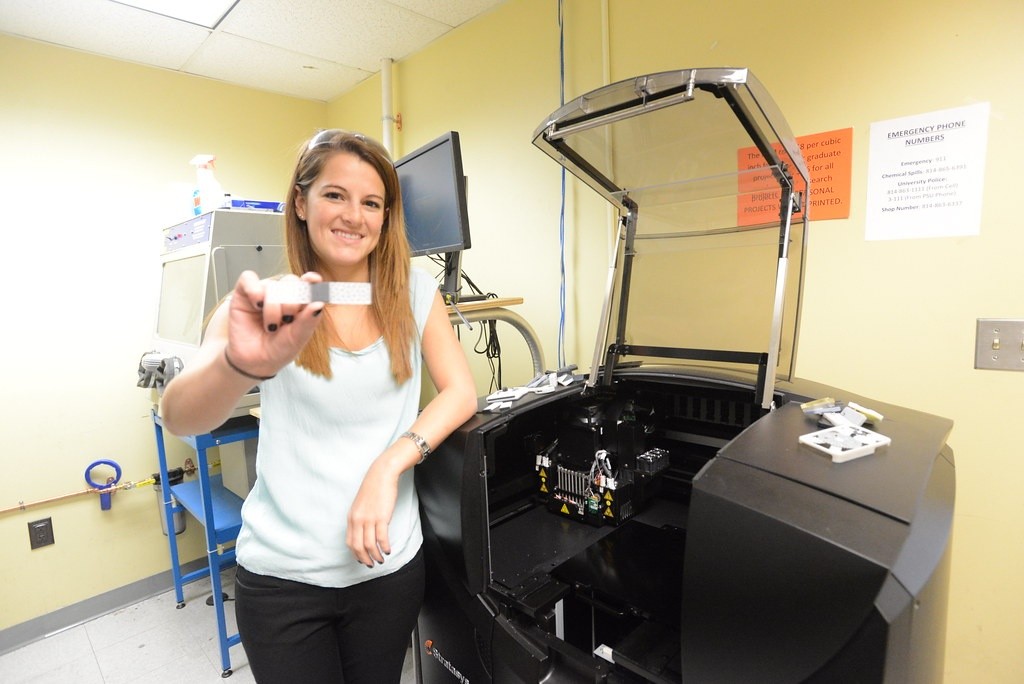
[162,129,479,683]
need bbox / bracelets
[224,346,277,381]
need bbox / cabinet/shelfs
[151,407,260,677]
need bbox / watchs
[399,430,431,464]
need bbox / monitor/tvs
[392,131,471,257]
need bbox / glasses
[309,131,394,172]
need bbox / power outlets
[27,517,56,550]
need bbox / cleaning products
[190,152,225,215]
